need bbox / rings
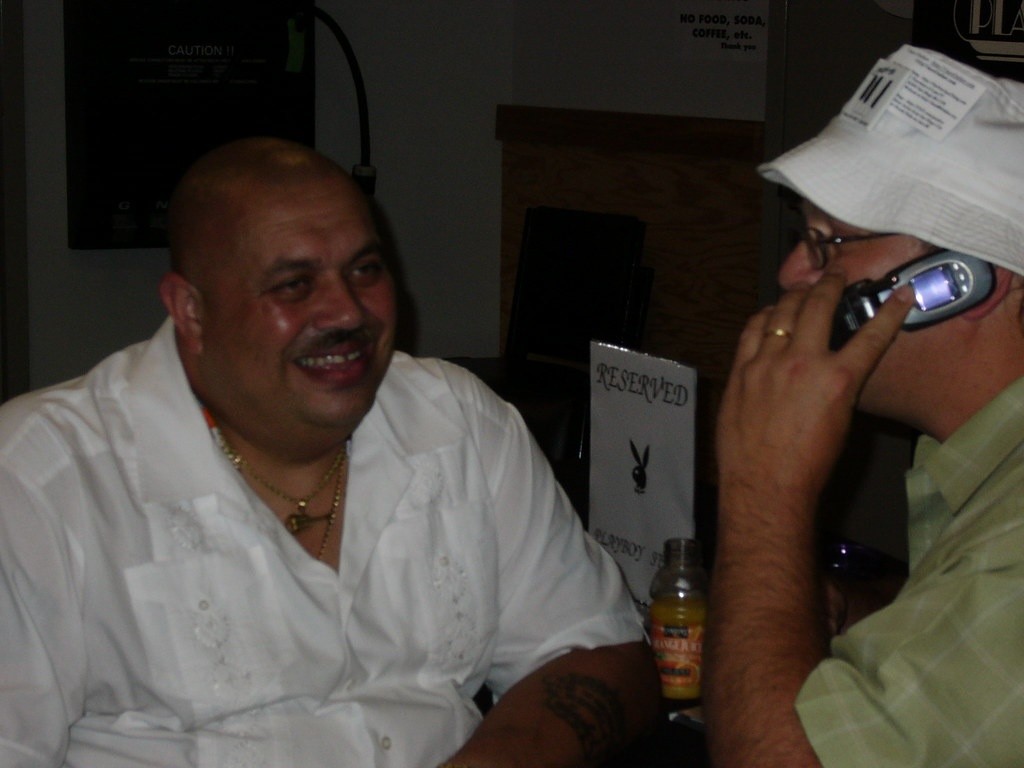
[762,327,791,341]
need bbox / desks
[443,357,908,768]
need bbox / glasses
[791,227,900,270]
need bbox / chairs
[506,197,930,574]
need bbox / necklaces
[314,455,343,562]
[206,407,346,535]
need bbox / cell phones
[828,248,998,354]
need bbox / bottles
[647,538,707,709]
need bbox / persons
[0,138,666,768]
[701,44,1024,767]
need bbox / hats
[756,43,1024,276]
